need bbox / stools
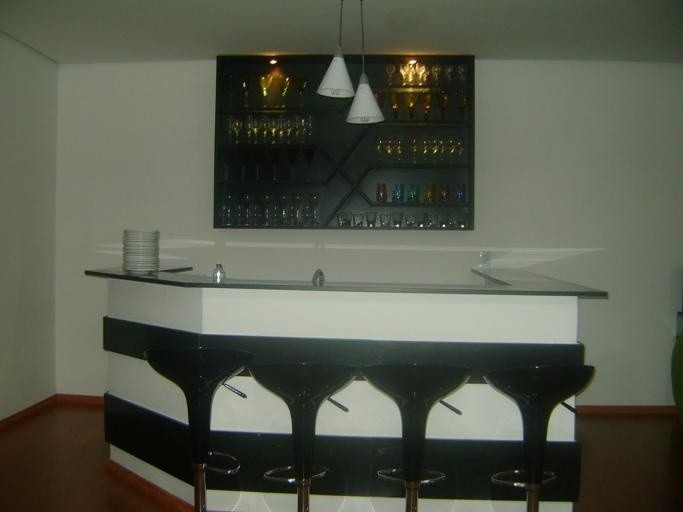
[144,346,252,512]
[247,362,360,512]
[483,365,595,512]
[361,363,472,512]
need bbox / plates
[122,230,159,277]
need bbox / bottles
[213,263,226,281]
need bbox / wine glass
[416,63,426,87]
[225,115,312,145]
[399,64,410,87]
[446,64,455,86]
[457,64,467,87]
[374,135,466,163]
[388,92,468,120]
[221,193,321,228]
[385,64,396,87]
[431,64,442,87]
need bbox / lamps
[316,0,355,98]
[346,0,385,124]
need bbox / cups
[390,213,404,227]
[380,214,391,228]
[392,184,403,202]
[428,215,440,230]
[440,184,448,203]
[417,214,427,228]
[405,214,417,227]
[352,214,364,227]
[376,182,387,202]
[455,184,465,205]
[423,184,435,203]
[335,212,352,227]
[365,212,377,227]
[407,184,418,204]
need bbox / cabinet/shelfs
[213,54,475,231]
